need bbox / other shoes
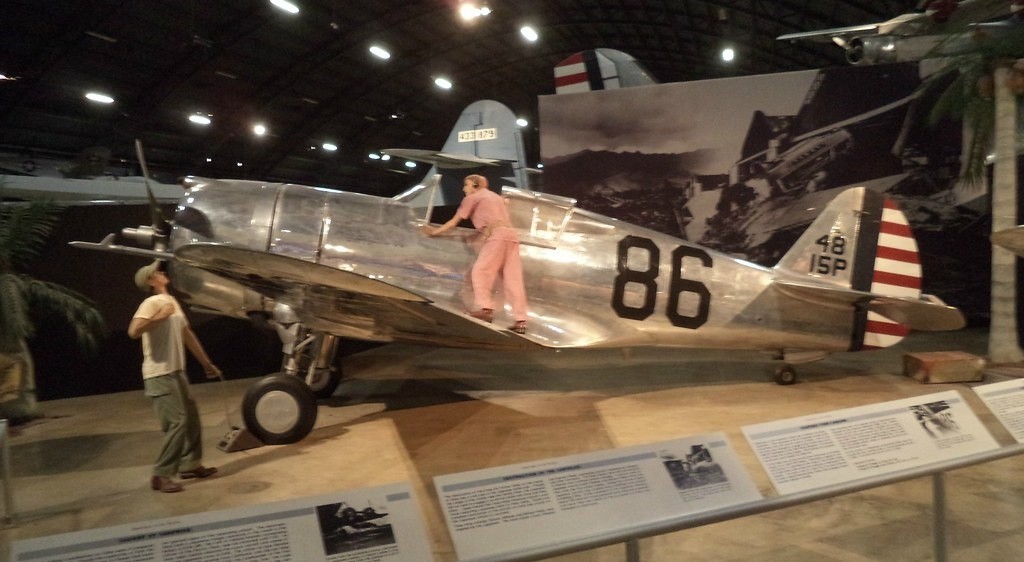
[470,309,493,323]
[509,324,525,334]
[179,467,217,478]
[152,476,181,493]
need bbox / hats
[135,259,161,290]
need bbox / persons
[421,175,529,334]
[128,260,221,492]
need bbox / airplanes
[730,83,927,193]
[63,96,971,447]
[773,0,1024,262]
